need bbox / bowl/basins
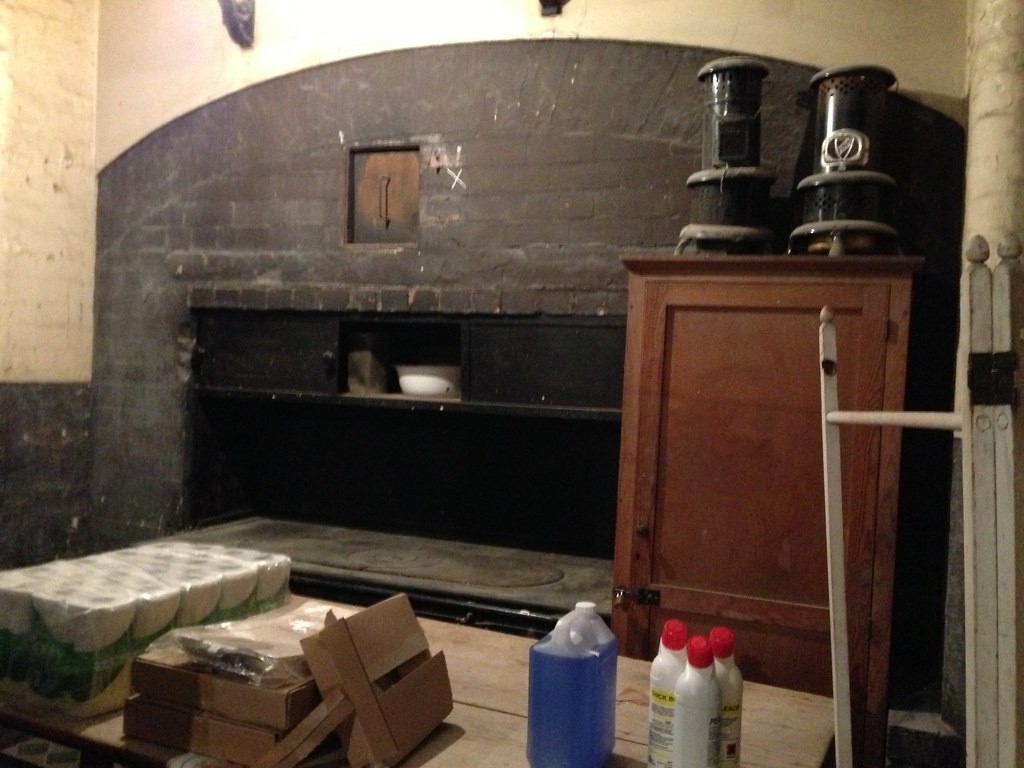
[392,362,461,397]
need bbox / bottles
[711,626,743,768]
[648,619,687,768]
[673,635,720,768]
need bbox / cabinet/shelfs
[608,252,924,768]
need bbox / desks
[0,591,835,768]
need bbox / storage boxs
[123,593,453,768]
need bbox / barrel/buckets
[525,603,617,768]
